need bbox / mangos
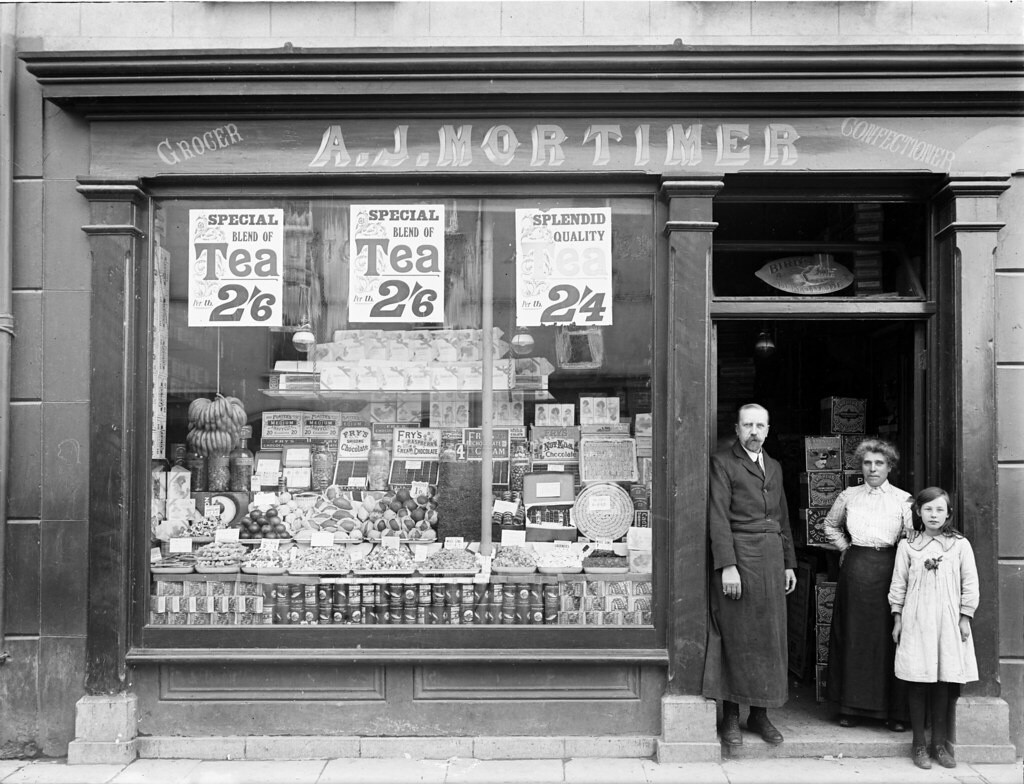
[306,489,355,534]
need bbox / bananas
[186,397,247,457]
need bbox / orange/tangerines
[236,510,289,540]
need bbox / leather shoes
[882,719,906,732]
[839,715,862,726]
[912,746,931,769]
[930,744,956,768]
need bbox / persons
[701,403,798,746]
[888,488,980,769]
[823,440,920,731]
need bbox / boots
[747,706,783,744]
[721,700,743,746]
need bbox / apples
[357,492,438,540]
